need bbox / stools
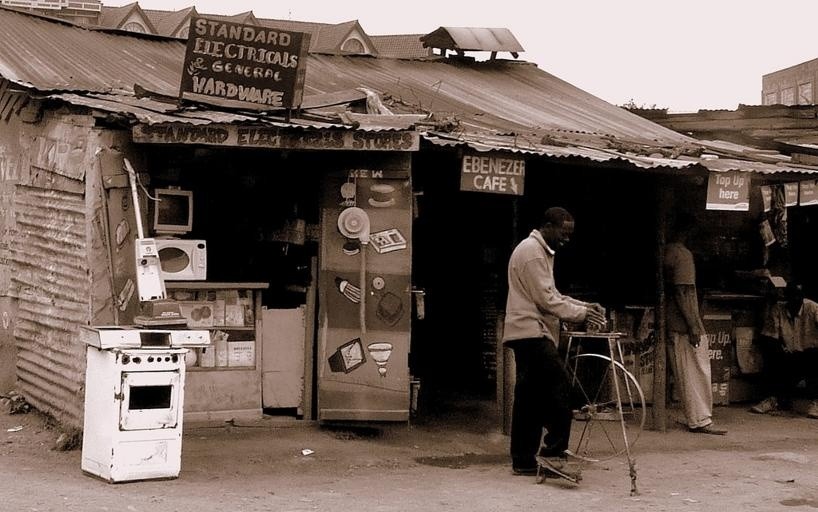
[535,332,644,493]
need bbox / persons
[500,206,604,481]
[664,215,728,434]
[752,286,818,417]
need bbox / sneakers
[535,452,584,484]
[750,397,777,415]
[512,465,562,479]
[806,400,818,420]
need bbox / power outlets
[369,226,407,255]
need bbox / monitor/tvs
[153,188,194,240]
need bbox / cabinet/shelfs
[136,279,268,420]
[82,346,190,483]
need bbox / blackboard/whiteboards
[179,17,303,108]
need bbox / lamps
[334,275,362,304]
[369,276,406,329]
[366,341,393,376]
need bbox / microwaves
[154,237,207,282]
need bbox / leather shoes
[690,423,729,435]
